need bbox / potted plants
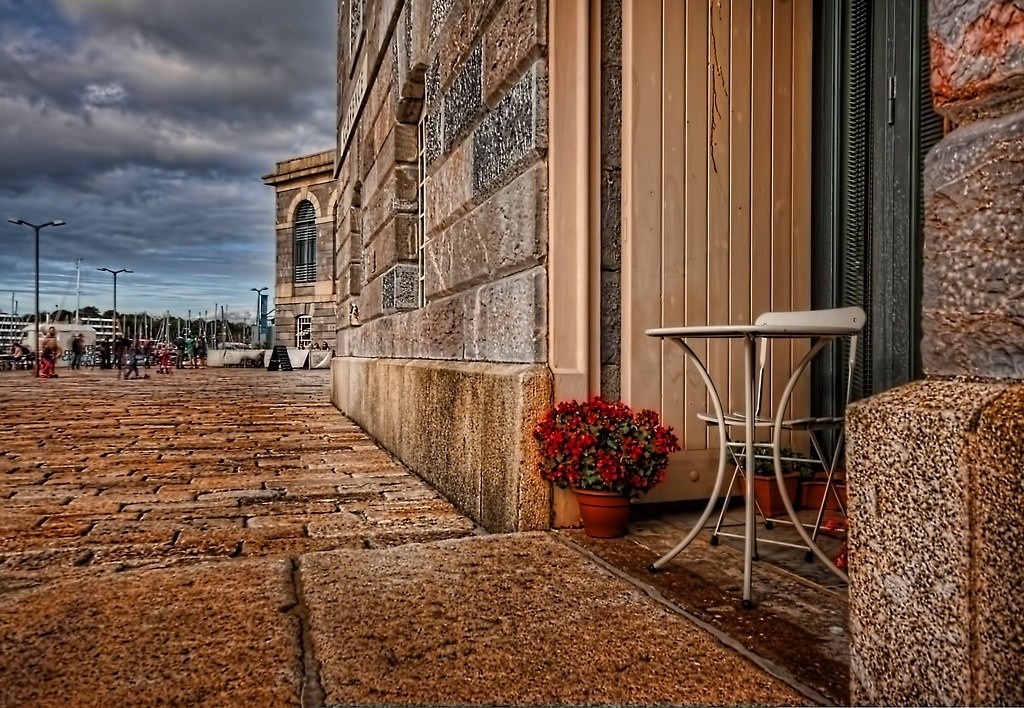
[727,439,812,518]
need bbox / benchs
[0,359,25,371]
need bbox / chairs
[710,306,865,565]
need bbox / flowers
[532,395,682,504]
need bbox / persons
[298,341,329,350]
[12,326,207,380]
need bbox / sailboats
[0,258,252,367]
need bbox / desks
[645,326,860,609]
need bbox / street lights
[251,287,269,345]
[97,267,134,370]
[7,216,67,378]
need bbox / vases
[572,486,631,539]
